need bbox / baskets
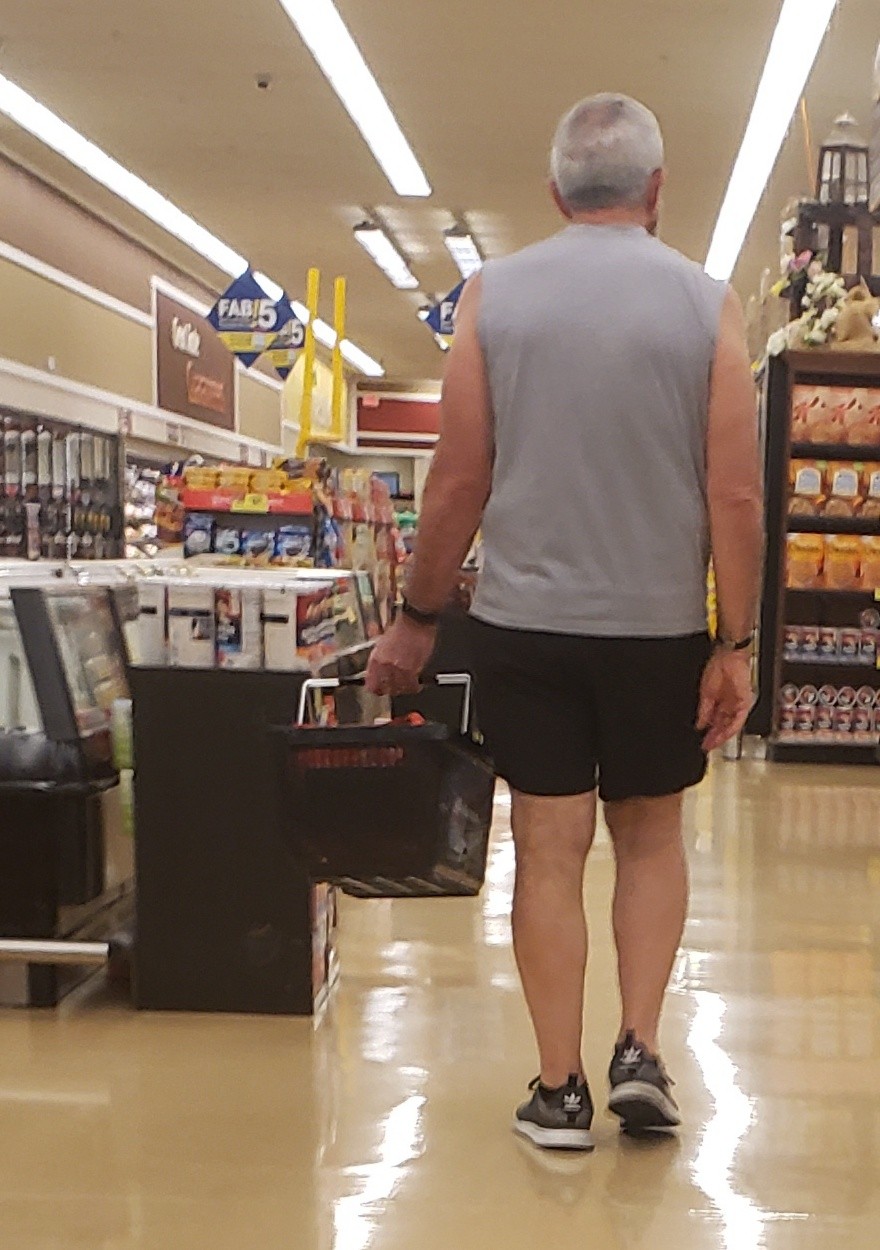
[260,674,497,899]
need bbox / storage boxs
[154,567,360,675]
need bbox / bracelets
[715,629,754,651]
[401,598,446,626]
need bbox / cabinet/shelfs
[739,344,879,770]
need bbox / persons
[367,92,763,1152]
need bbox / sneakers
[607,1027,682,1128]
[512,1073,596,1150]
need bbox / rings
[379,675,393,688]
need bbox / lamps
[442,226,483,281]
[353,222,419,289]
